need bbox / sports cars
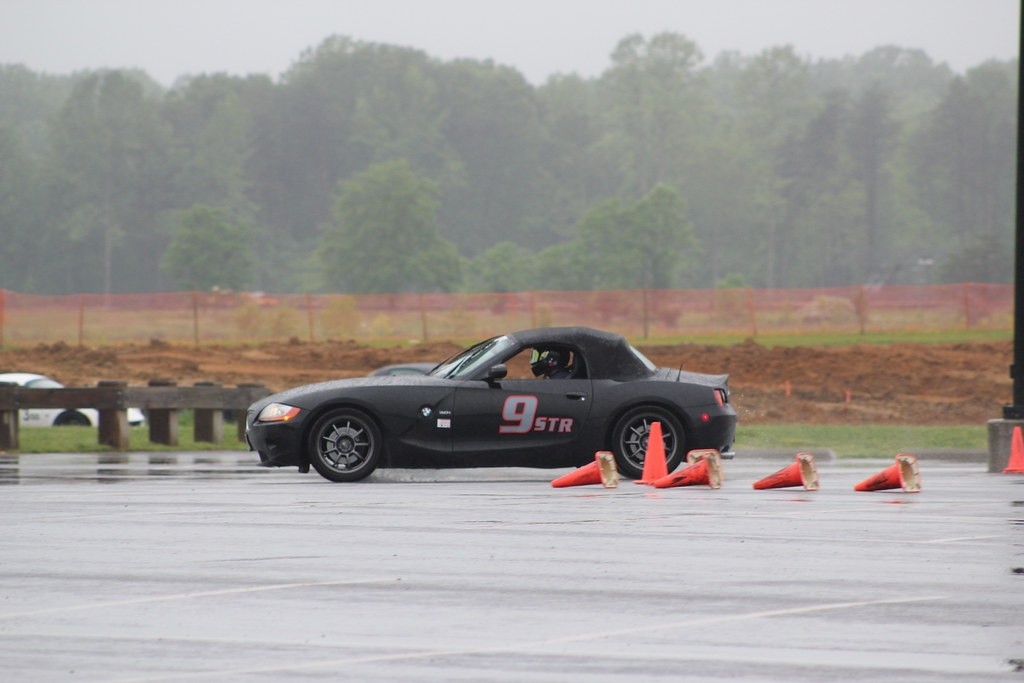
[246,326,738,483]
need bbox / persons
[530,346,575,379]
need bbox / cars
[0,371,147,429]
[368,363,440,378]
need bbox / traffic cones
[999,426,1024,474]
[654,452,722,490]
[753,451,822,492]
[635,421,669,485]
[853,454,923,494]
[550,451,619,489]
[686,449,721,467]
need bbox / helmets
[530,345,571,377]
